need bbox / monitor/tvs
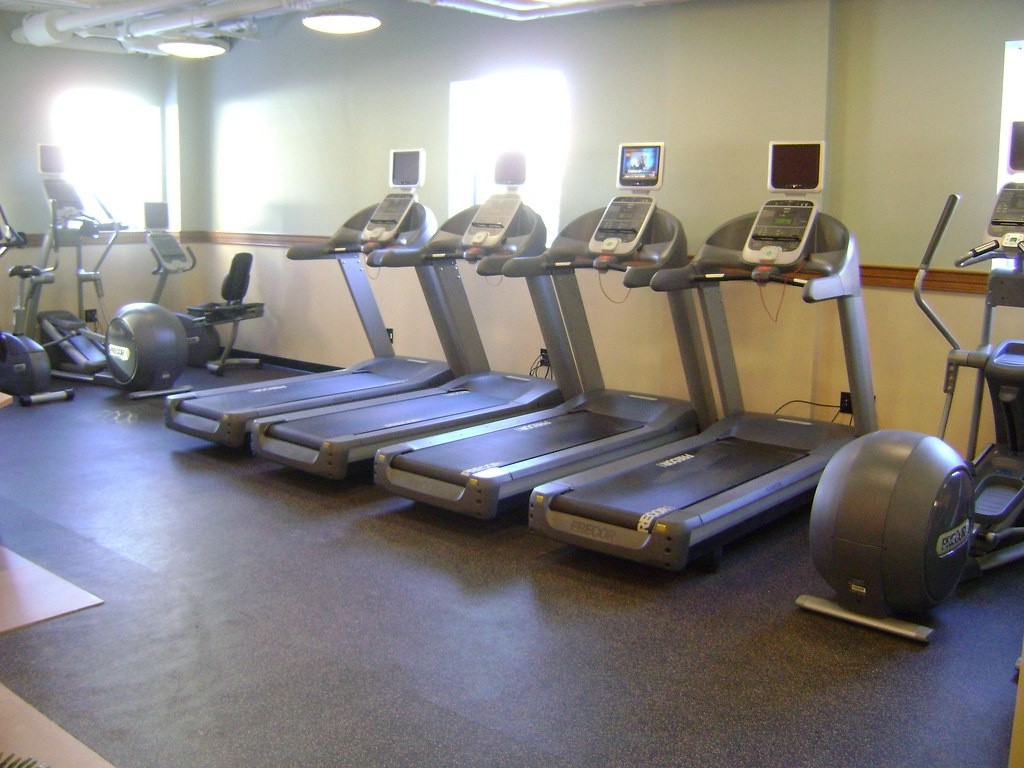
[389,149,426,189]
[1006,119,1024,176]
[144,202,169,230]
[616,142,665,190]
[495,150,527,185]
[768,140,825,194]
[37,144,64,174]
